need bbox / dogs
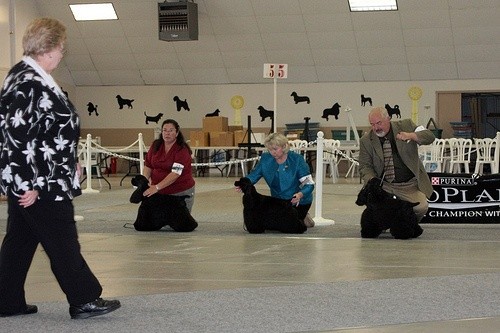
[130,175,198,232]
[356,178,423,240]
[234,177,311,234]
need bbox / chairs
[418,138,498,175]
[322,139,358,183]
[285,139,308,162]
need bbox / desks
[418,143,496,172]
[77,145,264,190]
[290,145,360,173]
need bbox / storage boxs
[190,116,299,147]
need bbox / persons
[136,119,196,229]
[359,106,435,234]
[235,132,315,231]
[0,18,121,320]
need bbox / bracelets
[155,185,160,191]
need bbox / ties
[383,137,395,183]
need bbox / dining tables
[226,147,249,178]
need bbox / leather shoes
[69,297,122,319]
[0,304,38,317]
